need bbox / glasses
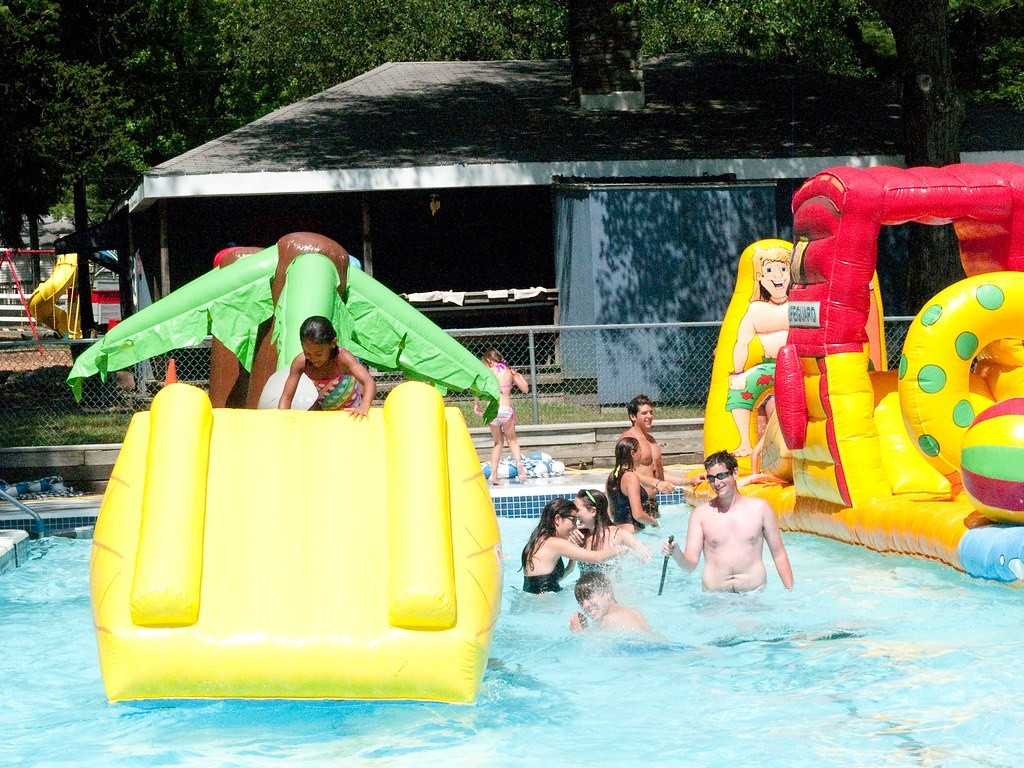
[560,514,577,524]
[706,471,732,482]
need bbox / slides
[27,253,83,339]
[88,380,504,703]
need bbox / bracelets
[655,479,662,490]
[651,519,658,527]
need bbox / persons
[603,437,663,535]
[515,498,631,595]
[569,570,656,646]
[472,348,529,486]
[663,449,794,594]
[277,315,377,421]
[614,394,699,528]
[756,395,778,441]
[569,489,652,586]
